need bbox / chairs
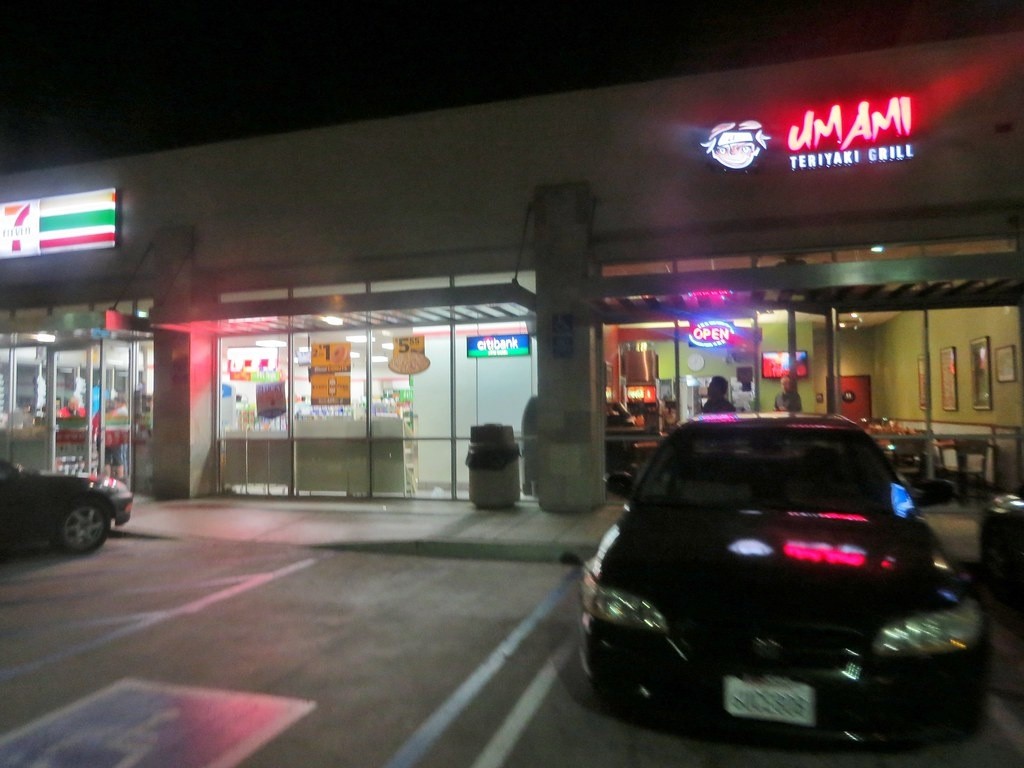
[947,439,989,502]
[890,436,923,474]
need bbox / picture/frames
[940,347,958,411]
[994,345,1017,382]
[968,336,992,410]
[918,355,930,411]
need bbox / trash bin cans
[468,424,521,509]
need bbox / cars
[978,480,1024,573]
[577,411,992,749]
[0,455,135,556]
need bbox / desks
[935,440,994,467]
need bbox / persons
[59,397,85,418]
[704,376,735,413]
[92,398,128,479]
[773,376,802,411]
[735,381,753,413]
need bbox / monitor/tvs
[761,350,809,379]
